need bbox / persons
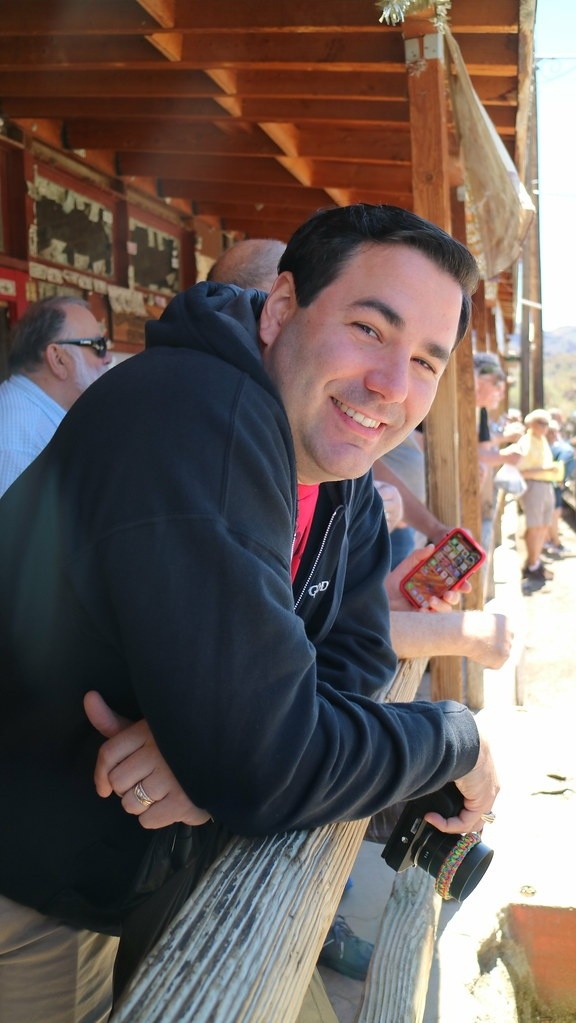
[0,204,515,1023]
[0,295,114,498]
[516,408,576,581]
[473,352,524,466]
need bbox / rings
[132,781,155,807]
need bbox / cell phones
[399,529,486,610]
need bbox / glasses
[41,336,107,358]
[538,421,549,426]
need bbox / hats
[524,408,551,425]
[473,354,507,381]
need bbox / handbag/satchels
[493,435,530,495]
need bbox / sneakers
[316,915,375,981]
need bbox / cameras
[379,780,494,905]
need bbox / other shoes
[544,540,552,548]
[524,565,554,580]
[554,545,570,553]
